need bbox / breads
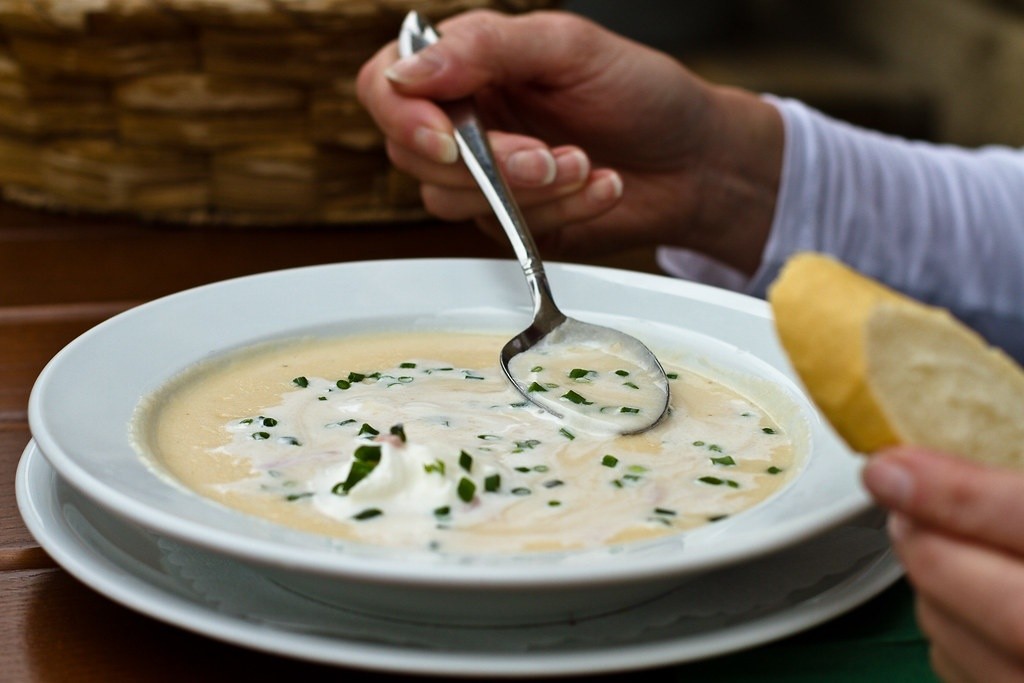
[768,250,1024,469]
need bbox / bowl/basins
[26,256,891,627]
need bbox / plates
[16,439,948,673]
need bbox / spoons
[398,8,670,435]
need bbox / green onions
[239,363,782,523]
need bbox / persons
[357,0,1024,683]
